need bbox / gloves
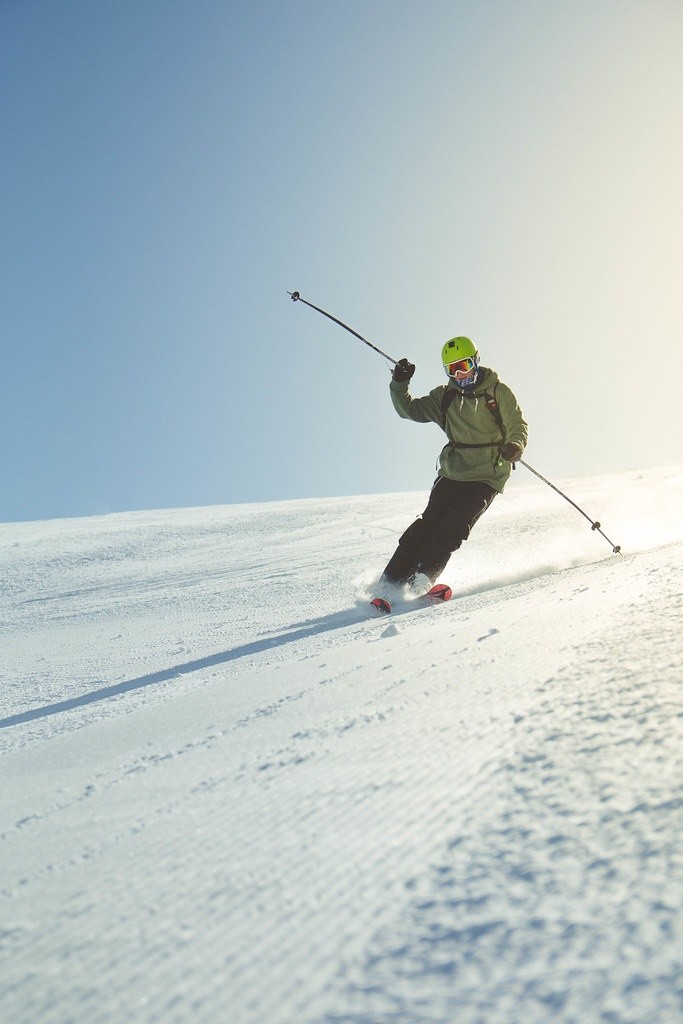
[502,443,521,463]
[392,358,416,382]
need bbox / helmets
[442,337,480,370]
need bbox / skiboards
[356,585,449,614]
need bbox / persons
[356,336,528,616]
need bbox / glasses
[444,357,475,377]
